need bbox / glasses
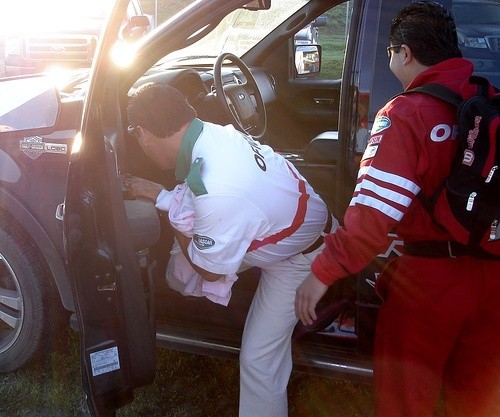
[128,125,150,136]
[387,44,416,59]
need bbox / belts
[403,239,481,258]
[300,208,338,254]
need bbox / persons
[294,2,500,417]
[121,83,339,417]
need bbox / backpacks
[388,75,500,261]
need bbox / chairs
[308,118,340,164]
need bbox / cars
[0,0,500,417]
